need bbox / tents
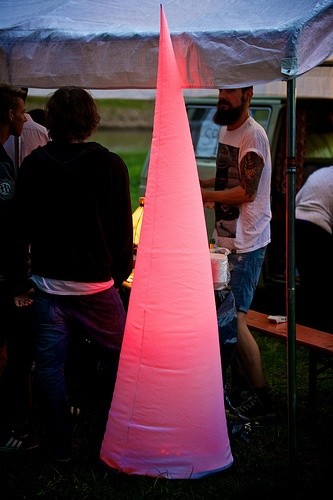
[0,1,333,439]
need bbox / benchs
[246,309,333,409]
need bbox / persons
[0,87,134,461]
[0,82,52,450]
[294,164,333,335]
[199,85,276,421]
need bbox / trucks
[136,55,333,241]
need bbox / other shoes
[0,429,40,453]
[236,393,277,420]
